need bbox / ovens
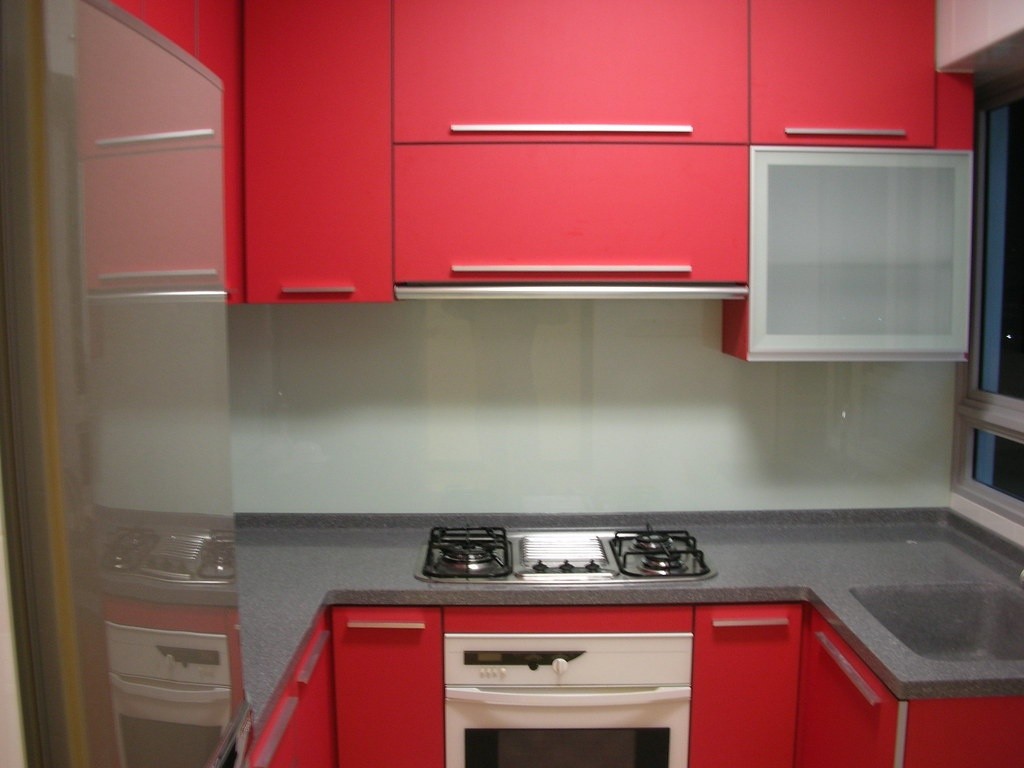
[443,632,693,768]
[105,623,232,768]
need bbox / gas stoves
[418,527,716,584]
[93,522,235,607]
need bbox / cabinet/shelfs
[239,599,1024,768]
[108,0,393,304]
[750,0,937,149]
[721,142,971,365]
[74,3,226,299]
[391,0,751,286]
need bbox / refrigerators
[0,0,257,768]
